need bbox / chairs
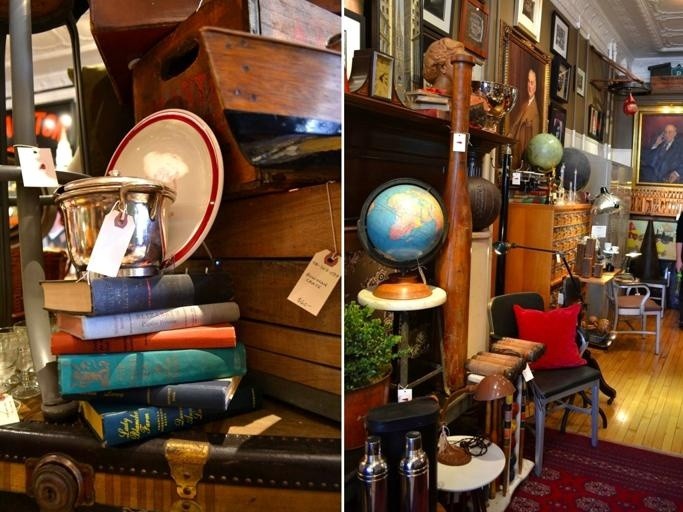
[485,290,601,471]
[606,278,666,354]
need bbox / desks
[573,267,622,320]
[344,92,520,237]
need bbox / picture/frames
[458,1,489,59]
[497,30,552,188]
[632,103,683,187]
[422,0,453,37]
[547,9,605,149]
[513,0,543,43]
[369,50,395,101]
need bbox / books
[408,87,451,120]
[38,269,261,451]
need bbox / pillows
[510,300,588,367]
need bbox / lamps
[588,185,624,214]
[496,239,589,311]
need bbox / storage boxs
[649,75,683,96]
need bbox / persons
[507,66,541,169]
[639,123,683,184]
[674,211,683,330]
[422,38,466,90]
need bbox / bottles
[396,430,431,512]
[582,257,593,278]
[356,436,391,512]
[593,255,604,279]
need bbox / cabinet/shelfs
[505,201,594,312]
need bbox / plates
[104,107,224,268]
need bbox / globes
[357,177,448,300]
[526,133,564,173]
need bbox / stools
[436,435,507,512]
[356,285,447,401]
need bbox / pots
[49,167,178,279]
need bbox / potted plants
[344,300,413,453]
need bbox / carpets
[496,420,683,512]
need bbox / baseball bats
[436,54,475,391]
